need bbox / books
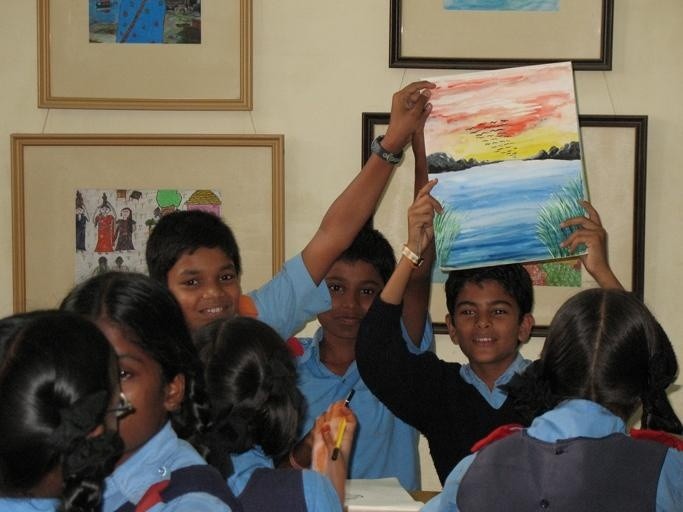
[344,476,424,512]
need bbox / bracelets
[291,431,314,468]
[371,135,403,164]
[401,243,424,268]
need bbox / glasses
[106,392,132,418]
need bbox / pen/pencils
[346,389,356,408]
[331,413,347,458]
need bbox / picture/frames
[361,111,648,339]
[389,0,614,71]
[36,0,253,111]
[10,133,285,316]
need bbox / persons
[174,316,358,512]
[59,271,243,512]
[145,80,436,343]
[288,94,436,493]
[354,178,683,487]
[0,310,132,512]
[419,287,682,512]
